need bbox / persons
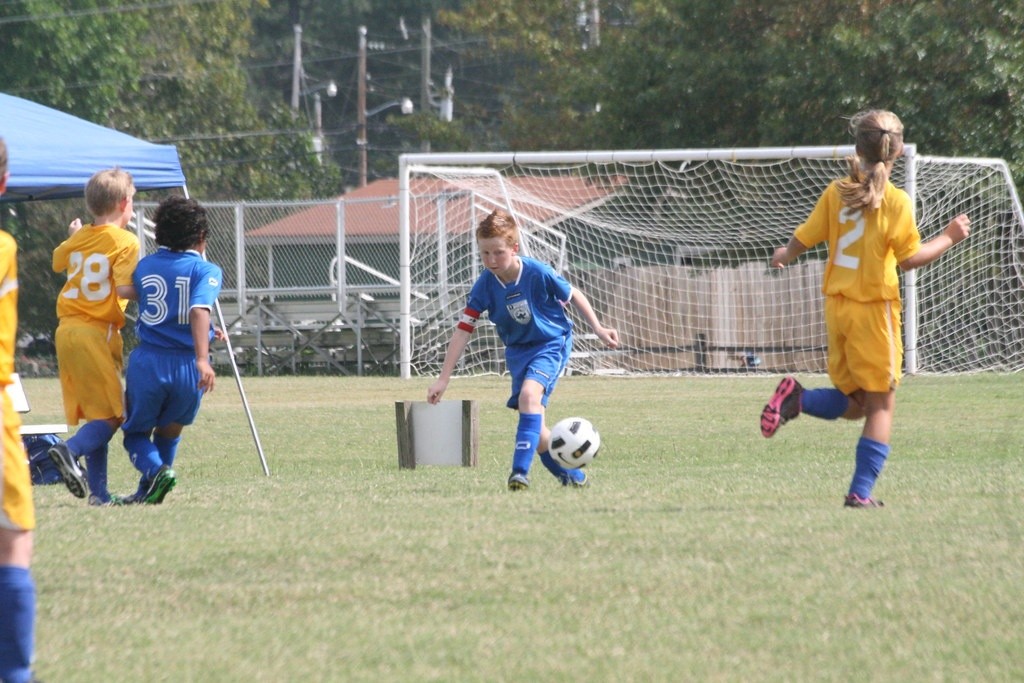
[0,136,41,683]
[426,206,618,491]
[124,194,223,504]
[47,167,142,509]
[756,110,969,514]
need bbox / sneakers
[47,439,89,499]
[508,472,529,492]
[141,463,177,506]
[761,376,806,438]
[843,493,885,509]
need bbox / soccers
[548,416,602,470]
